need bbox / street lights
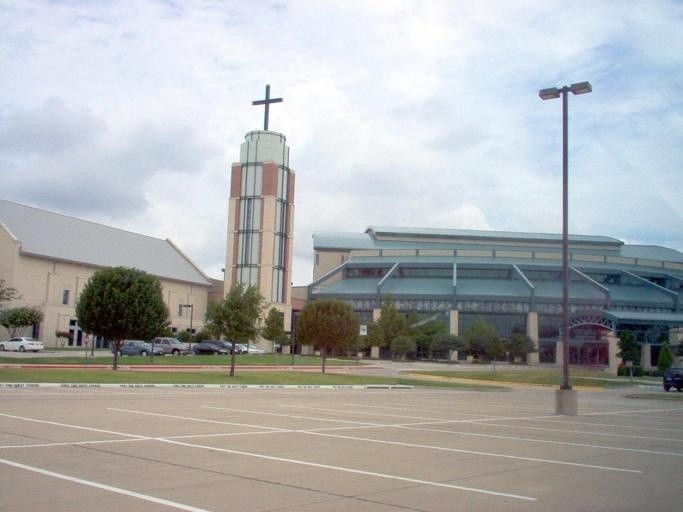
[183,304,193,354]
[537,79,593,420]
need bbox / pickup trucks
[146,335,188,357]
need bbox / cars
[661,355,683,392]
[0,335,45,353]
[190,339,259,356]
[108,338,165,357]
